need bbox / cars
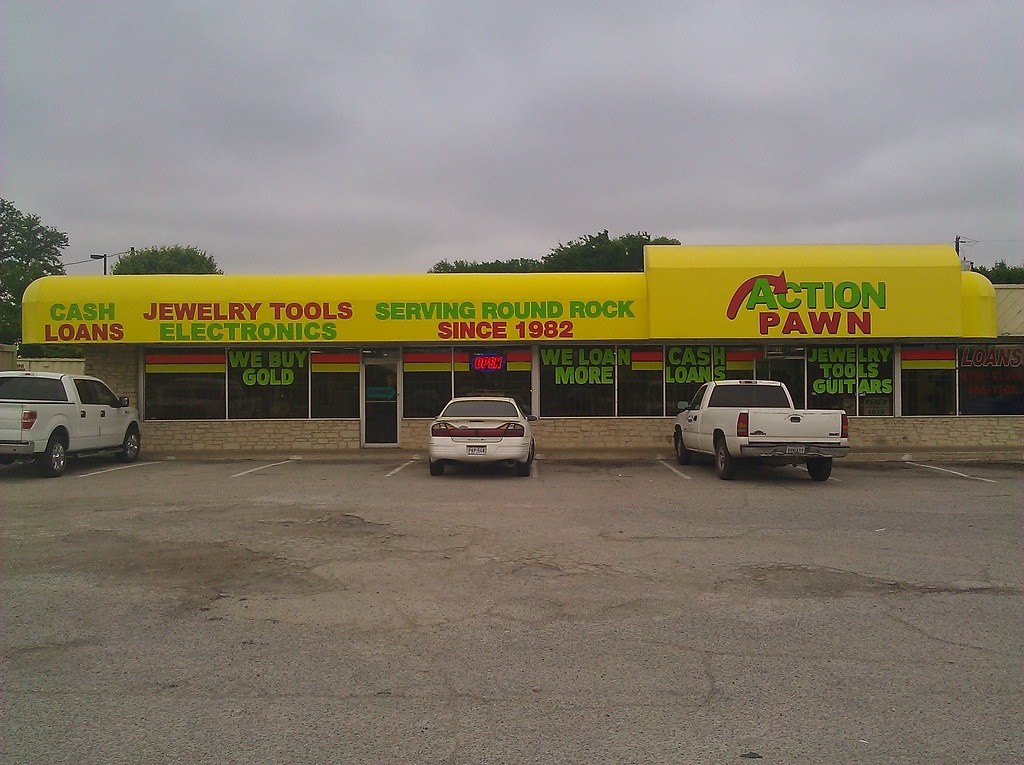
[429,396,537,477]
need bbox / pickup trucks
[0,370,141,477]
[673,380,850,481]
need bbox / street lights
[90,252,108,275]
